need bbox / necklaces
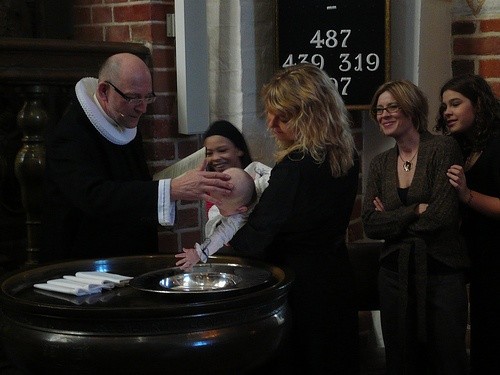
[397,151,418,172]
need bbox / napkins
[33,271,134,297]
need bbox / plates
[127,263,271,294]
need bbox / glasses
[373,104,402,115]
[105,80,157,106]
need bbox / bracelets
[468,188,474,204]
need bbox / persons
[362,78,468,375]
[202,119,253,213]
[434,75,500,375]
[174,166,273,270]
[47,52,234,259]
[216,64,360,375]
